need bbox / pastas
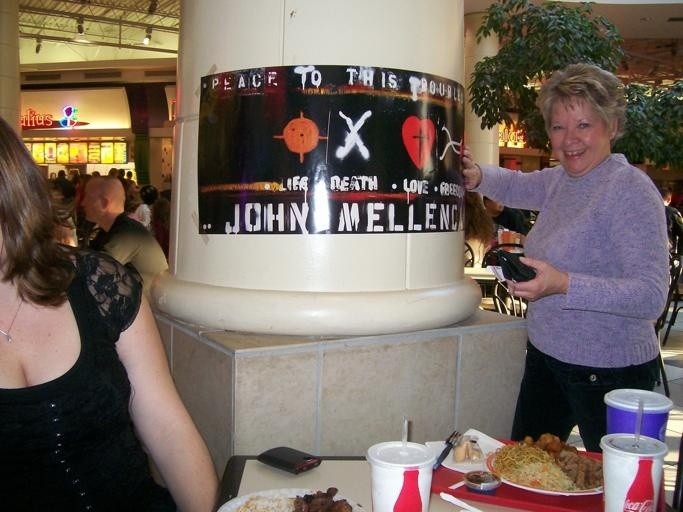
[491,440,551,480]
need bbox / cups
[364,440,435,512]
[598,432,668,512]
[602,387,675,443]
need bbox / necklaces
[0,296,23,342]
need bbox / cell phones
[258,446,321,474]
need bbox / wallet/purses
[497,249,537,282]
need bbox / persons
[658,185,683,264]
[462,60,671,456]
[483,194,528,237]
[48,166,172,291]
[0,115,222,512]
[464,190,495,269]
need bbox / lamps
[143,27,153,44]
[76,18,85,33]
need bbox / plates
[483,442,605,496]
[217,487,369,512]
[424,427,509,476]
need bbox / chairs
[464,242,527,318]
[656,253,683,396]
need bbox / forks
[431,429,462,472]
